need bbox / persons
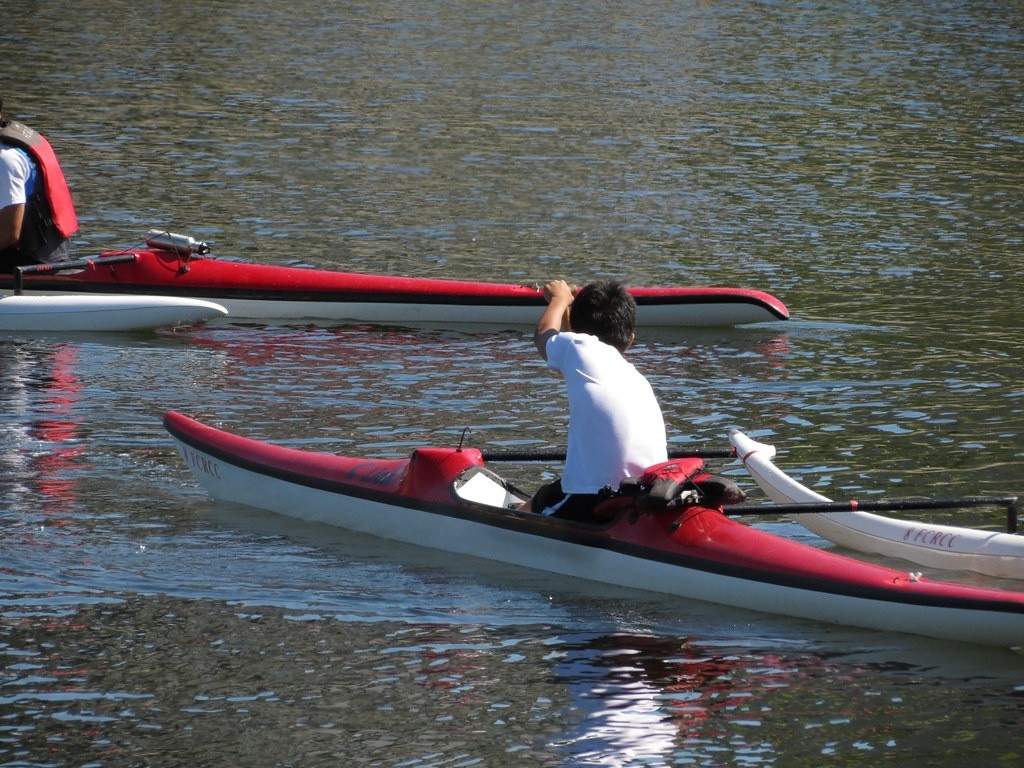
[508,280,669,524]
[0,101,78,276]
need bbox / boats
[724,426,1024,582]
[0,240,790,336]
[162,407,1024,650]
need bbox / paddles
[484,445,737,463]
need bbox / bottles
[145,229,210,255]
